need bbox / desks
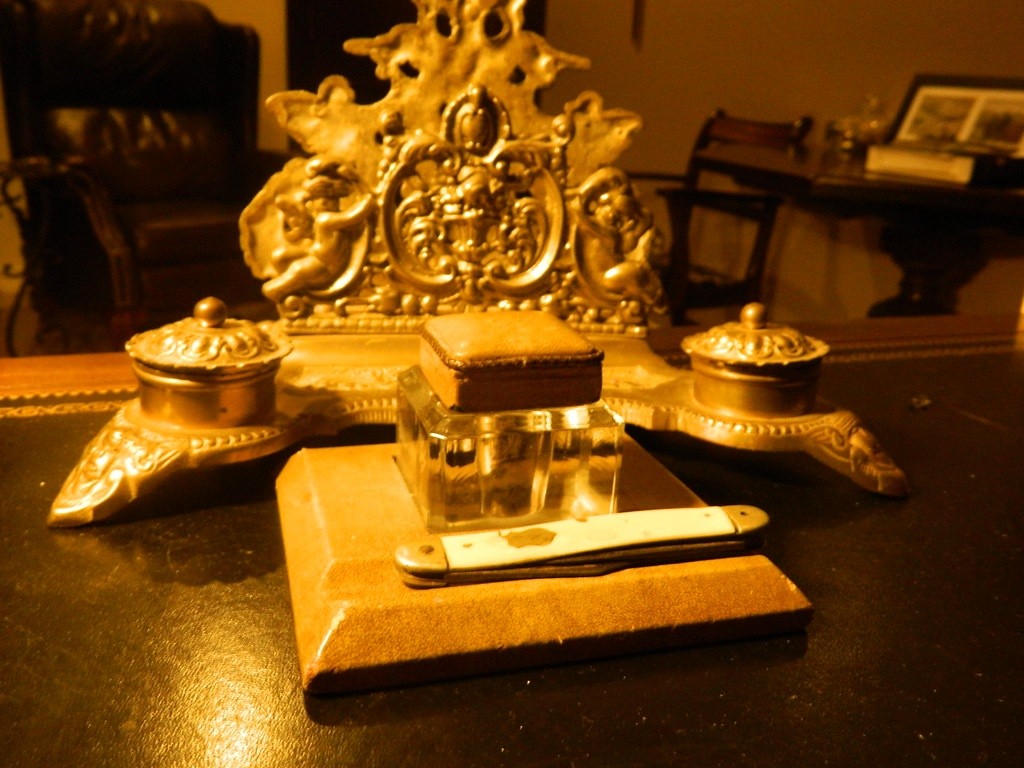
[696,146,1024,316]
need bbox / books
[864,137,1007,183]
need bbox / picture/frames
[883,70,1024,169]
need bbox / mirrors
[0,0,1024,418]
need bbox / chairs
[623,107,812,311]
[0,0,311,355]
